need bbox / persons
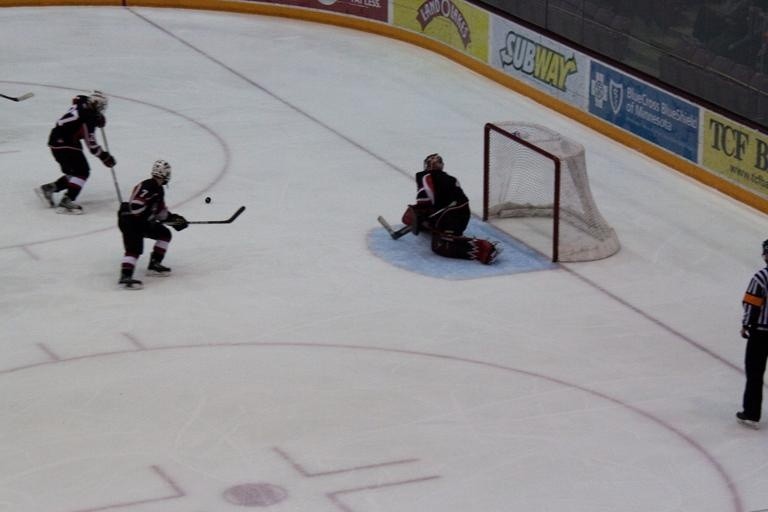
[735,237,767,422]
[402,153,497,265]
[115,160,188,285]
[42,90,116,211]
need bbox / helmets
[423,154,443,171]
[151,160,172,180]
[88,90,107,111]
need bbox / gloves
[101,151,116,167]
[169,214,188,231]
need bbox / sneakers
[148,262,171,273]
[737,411,761,423]
[118,278,143,286]
[57,197,82,209]
[41,184,54,207]
